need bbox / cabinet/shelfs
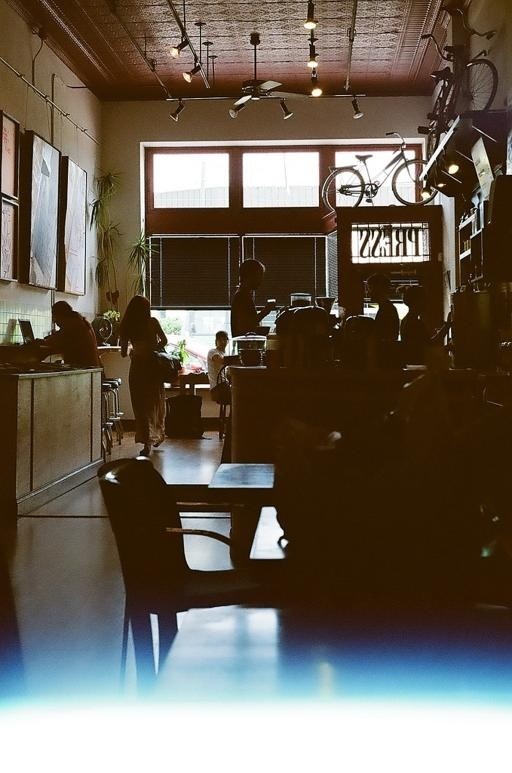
[454,176,504,293]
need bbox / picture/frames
[0,108,89,300]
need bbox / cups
[238,347,264,365]
[266,298,277,309]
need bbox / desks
[0,341,131,365]
[178,373,209,396]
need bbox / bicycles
[317,127,437,206]
[413,32,499,155]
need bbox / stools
[100,376,125,463]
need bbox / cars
[162,340,210,380]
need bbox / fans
[91,316,113,346]
[162,27,369,103]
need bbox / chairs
[216,362,235,441]
[98,453,286,688]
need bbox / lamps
[302,0,324,98]
[170,0,207,83]
[166,92,369,122]
[434,176,449,188]
[441,159,463,178]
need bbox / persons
[368,273,399,340]
[119,296,168,456]
[206,331,232,389]
[24,297,106,380]
[231,257,273,342]
[397,282,449,340]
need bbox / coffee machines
[289,291,311,306]
[314,296,334,339]
